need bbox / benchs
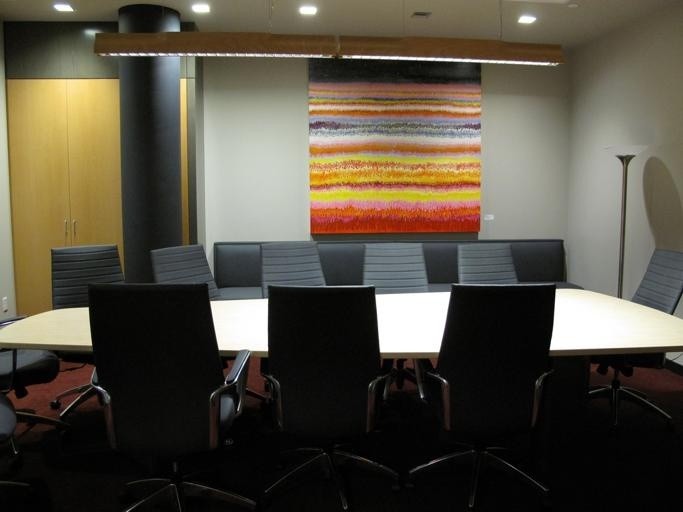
[214,239,566,289]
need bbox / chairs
[457,242,519,284]
[0,313,58,462]
[150,243,225,301]
[587,249,683,428]
[261,241,326,298]
[362,242,429,293]
[45,285,563,512]
[50,244,126,420]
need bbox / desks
[0,285,683,355]
